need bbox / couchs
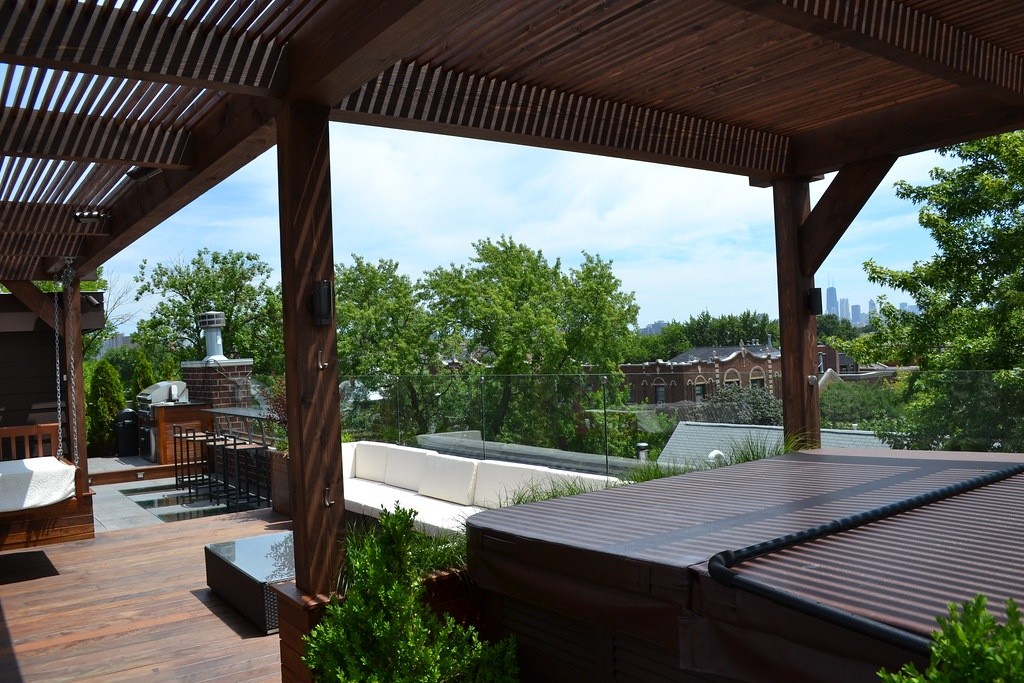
[338,441,631,544]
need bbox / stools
[171,423,272,508]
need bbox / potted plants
[265,422,290,517]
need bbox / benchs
[1,424,97,553]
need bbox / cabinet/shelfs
[148,402,213,464]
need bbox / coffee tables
[204,530,297,636]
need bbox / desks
[200,405,288,448]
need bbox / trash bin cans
[136,379,190,464]
